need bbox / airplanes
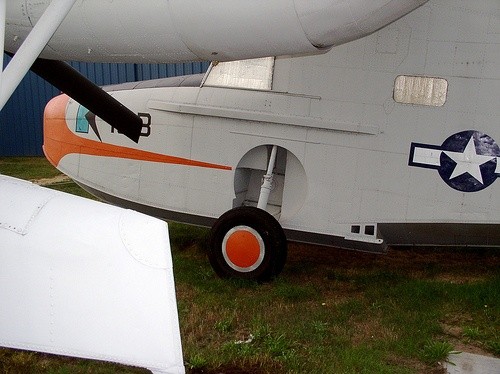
[1,0,499,288]
